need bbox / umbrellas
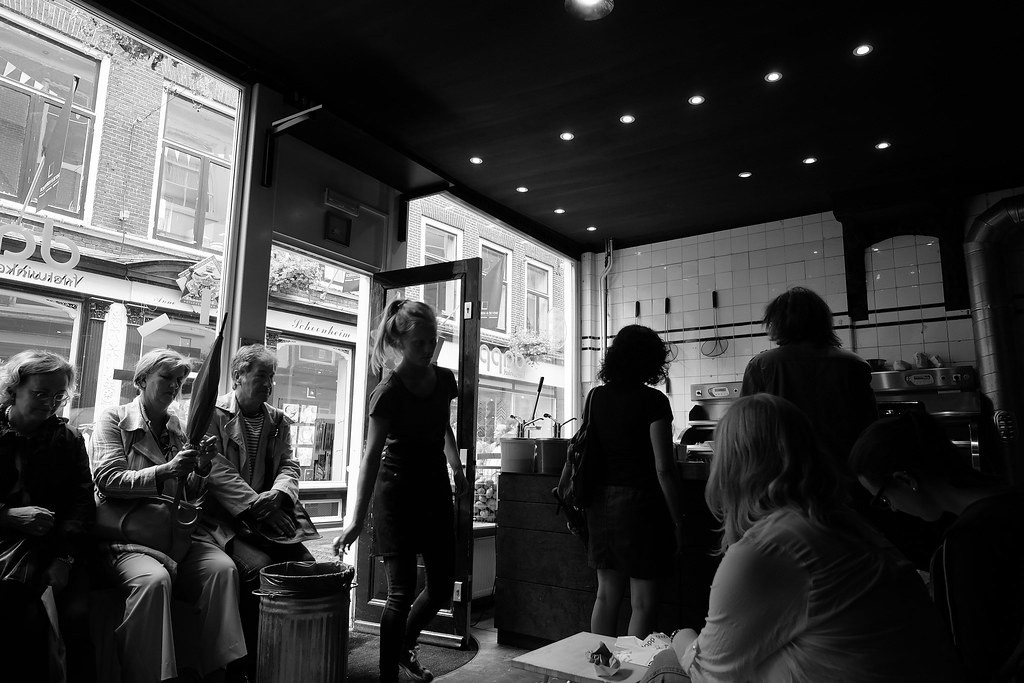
[170,311,229,526]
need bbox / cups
[968,424,980,453]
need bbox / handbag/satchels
[94,494,201,563]
[556,386,598,550]
[229,499,322,549]
[0,525,46,585]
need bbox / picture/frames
[323,211,352,247]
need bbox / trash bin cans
[251,558,359,683]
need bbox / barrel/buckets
[500,438,536,472]
[536,438,572,473]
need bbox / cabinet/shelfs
[495,472,629,651]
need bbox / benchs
[511,632,654,683]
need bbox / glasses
[20,386,69,402]
[870,482,889,511]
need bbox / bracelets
[670,628,683,643]
[453,464,463,478]
[673,512,687,529]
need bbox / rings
[39,527,43,532]
[680,552,684,555]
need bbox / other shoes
[399,645,434,682]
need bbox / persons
[848,417,1024,683]
[638,394,928,683]
[93,348,252,683]
[0,350,128,683]
[201,344,316,642]
[334,299,469,683]
[581,325,689,640]
[740,286,876,476]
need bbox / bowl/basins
[866,359,886,372]
